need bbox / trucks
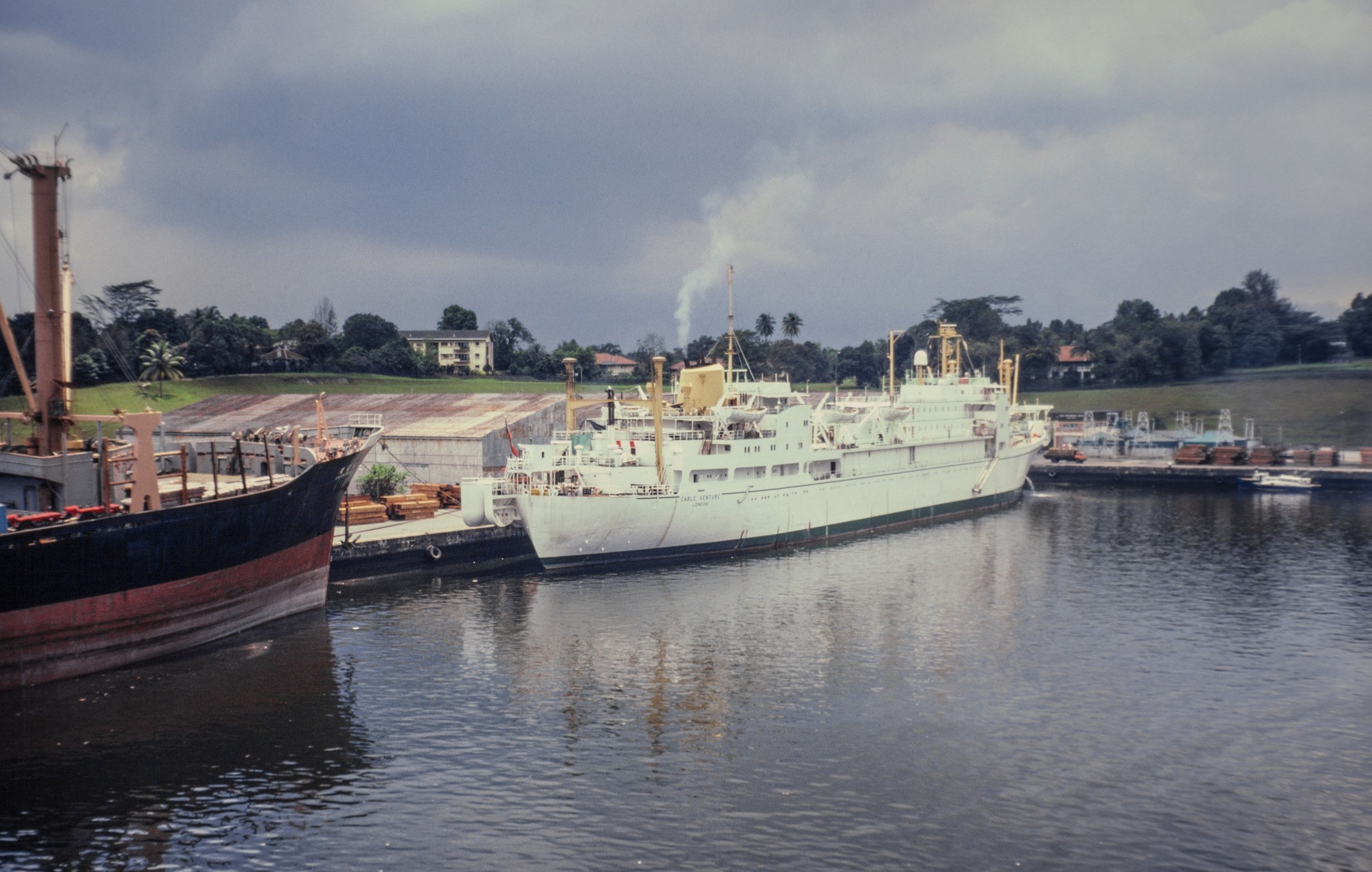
[1043,447,1086,463]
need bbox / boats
[0,125,393,690]
[509,260,1055,574]
